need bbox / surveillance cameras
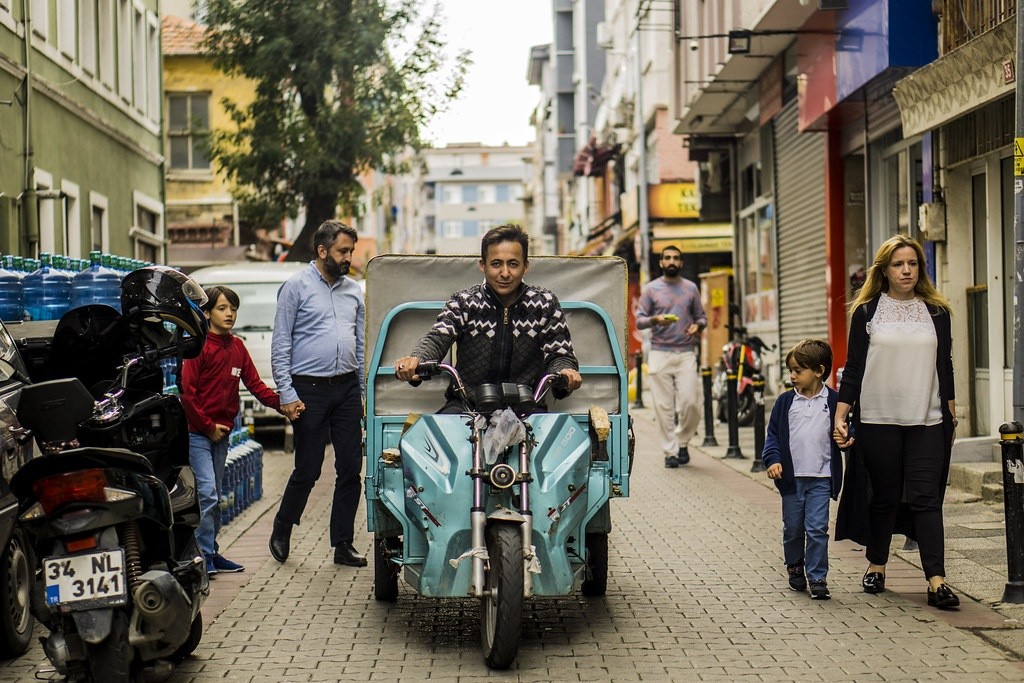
[690,41,699,50]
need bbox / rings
[398,365,404,370]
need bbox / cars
[180,261,321,445]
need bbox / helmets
[121,266,207,359]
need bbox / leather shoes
[334,541,368,566]
[927,584,959,608]
[269,512,293,561]
[862,563,886,592]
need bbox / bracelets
[953,418,958,428]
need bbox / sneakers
[205,554,217,573]
[213,553,245,572]
[809,580,831,599]
[787,562,808,591]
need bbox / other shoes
[665,457,679,469]
[677,447,690,464]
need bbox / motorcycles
[0,260,210,683]
[715,303,778,428]
[362,256,636,672]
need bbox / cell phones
[664,314,679,321]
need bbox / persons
[179,286,304,575]
[835,234,959,608]
[635,245,707,468]
[396,224,583,414]
[762,340,856,599]
[269,220,368,566]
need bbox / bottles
[0,251,190,335]
[222,409,263,523]
[160,356,176,388]
[163,385,180,401]
